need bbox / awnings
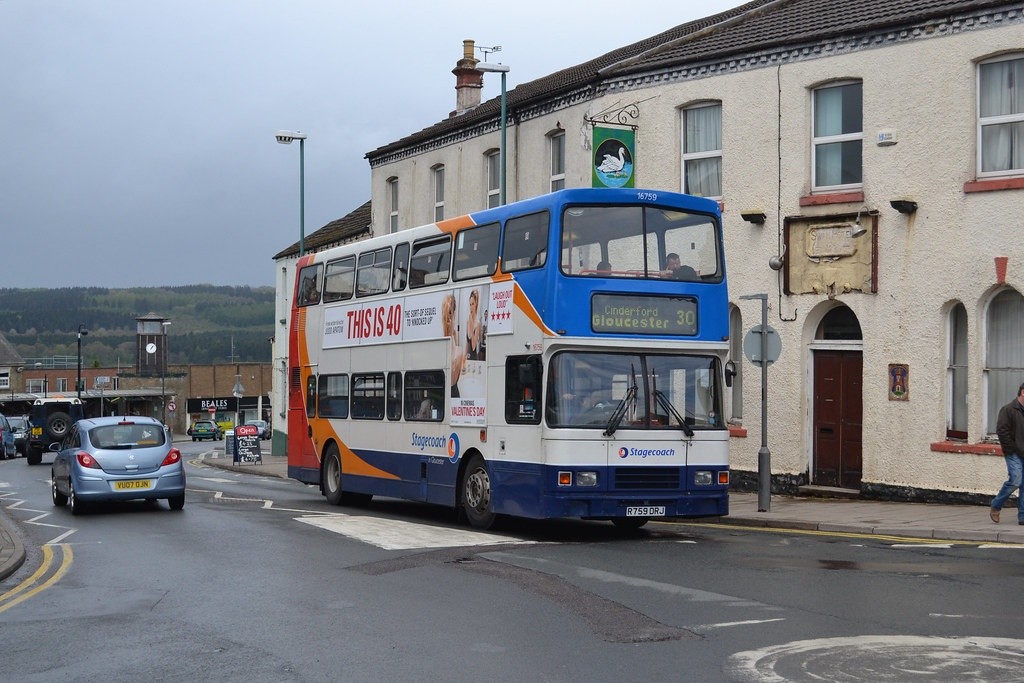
[0,389,179,401]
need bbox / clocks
[146,343,156,353]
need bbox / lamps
[769,244,786,271]
[851,205,882,239]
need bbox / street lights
[75,323,89,399]
[160,320,172,423]
[273,128,307,257]
[474,61,511,205]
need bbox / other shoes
[1019,521,1024,525]
[990,506,1000,523]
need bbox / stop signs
[208,406,216,413]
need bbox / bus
[286,187,732,533]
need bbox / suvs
[24,397,85,465]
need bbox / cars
[192,419,225,441]
[246,419,271,440]
[0,414,34,460]
[51,414,186,514]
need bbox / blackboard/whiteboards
[224,428,234,458]
[232,424,263,465]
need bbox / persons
[463,289,480,360]
[660,253,697,277]
[549,366,590,408]
[989,383,1024,527]
[441,294,465,398]
[306,288,321,303]
[597,261,612,275]
[478,310,488,360]
